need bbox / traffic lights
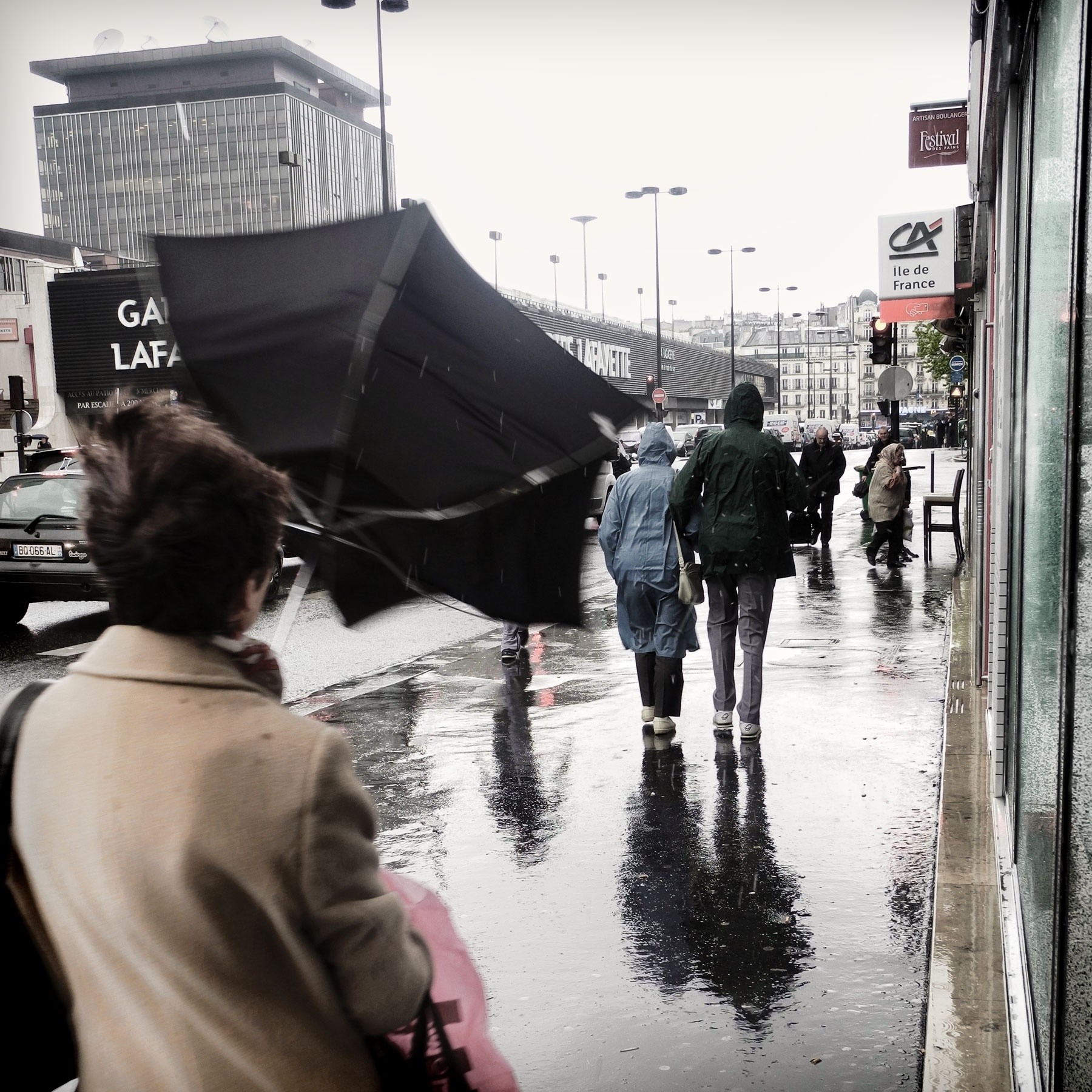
[869,317,892,364]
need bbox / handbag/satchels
[678,561,705,606]
[363,990,473,1091]
[379,867,522,1092]
[902,508,914,542]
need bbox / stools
[923,467,966,562]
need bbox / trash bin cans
[854,466,876,521]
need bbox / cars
[0,444,118,627]
[617,429,641,462]
[642,422,785,458]
[589,461,617,527]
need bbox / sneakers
[653,716,676,734]
[641,705,655,722]
[739,718,761,738]
[713,710,734,728]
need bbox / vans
[762,413,805,451]
[797,417,937,452]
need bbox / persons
[0,386,433,1092]
[667,382,807,740]
[862,426,906,482]
[865,443,910,567]
[799,427,847,547]
[501,621,532,658]
[934,414,956,449]
[598,420,700,735]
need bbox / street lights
[792,311,826,419]
[279,151,303,229]
[708,244,756,389]
[489,231,502,291]
[638,288,643,331]
[816,330,845,419]
[571,215,598,308]
[321,0,411,213]
[834,341,860,423]
[624,187,687,419]
[759,284,798,413]
[550,256,560,309]
[598,273,608,322]
[806,359,823,418]
[668,300,677,341]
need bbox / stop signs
[652,388,666,404]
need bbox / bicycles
[961,435,968,459]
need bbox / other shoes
[517,627,525,633]
[865,550,876,566]
[887,561,906,568]
[501,648,517,658]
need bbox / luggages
[788,510,812,547]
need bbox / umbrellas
[930,412,949,420]
[852,481,869,498]
[45,209,651,655]
